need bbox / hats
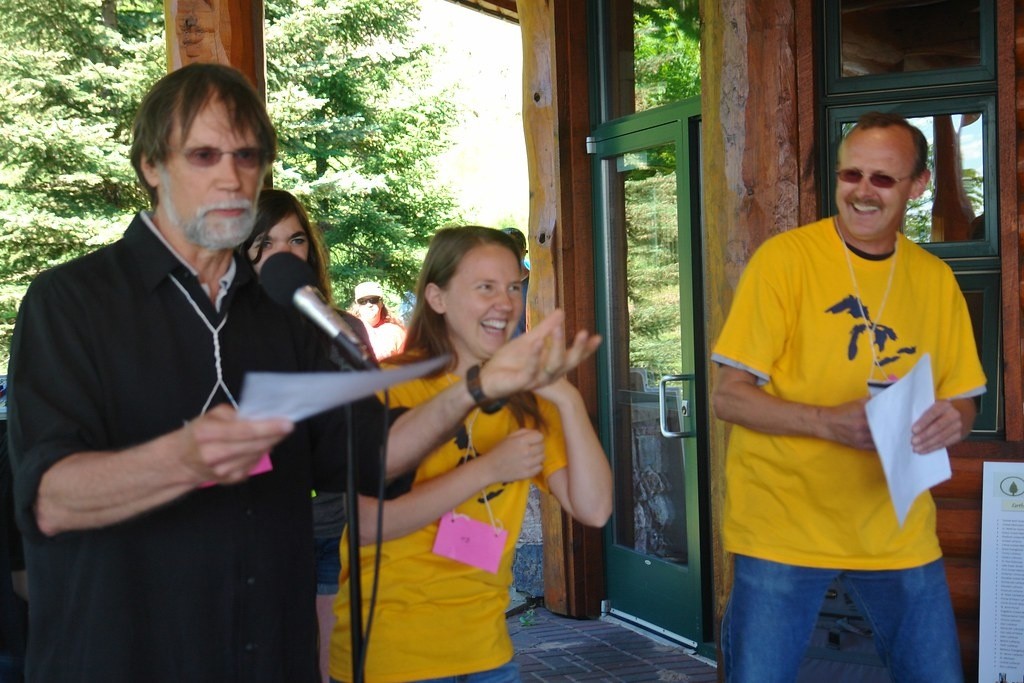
[354,282,383,303]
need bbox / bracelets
[466,365,506,414]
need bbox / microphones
[260,251,378,370]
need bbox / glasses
[833,167,913,190]
[358,297,379,305]
[169,146,263,168]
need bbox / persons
[710,112,987,683]
[6,64,615,683]
